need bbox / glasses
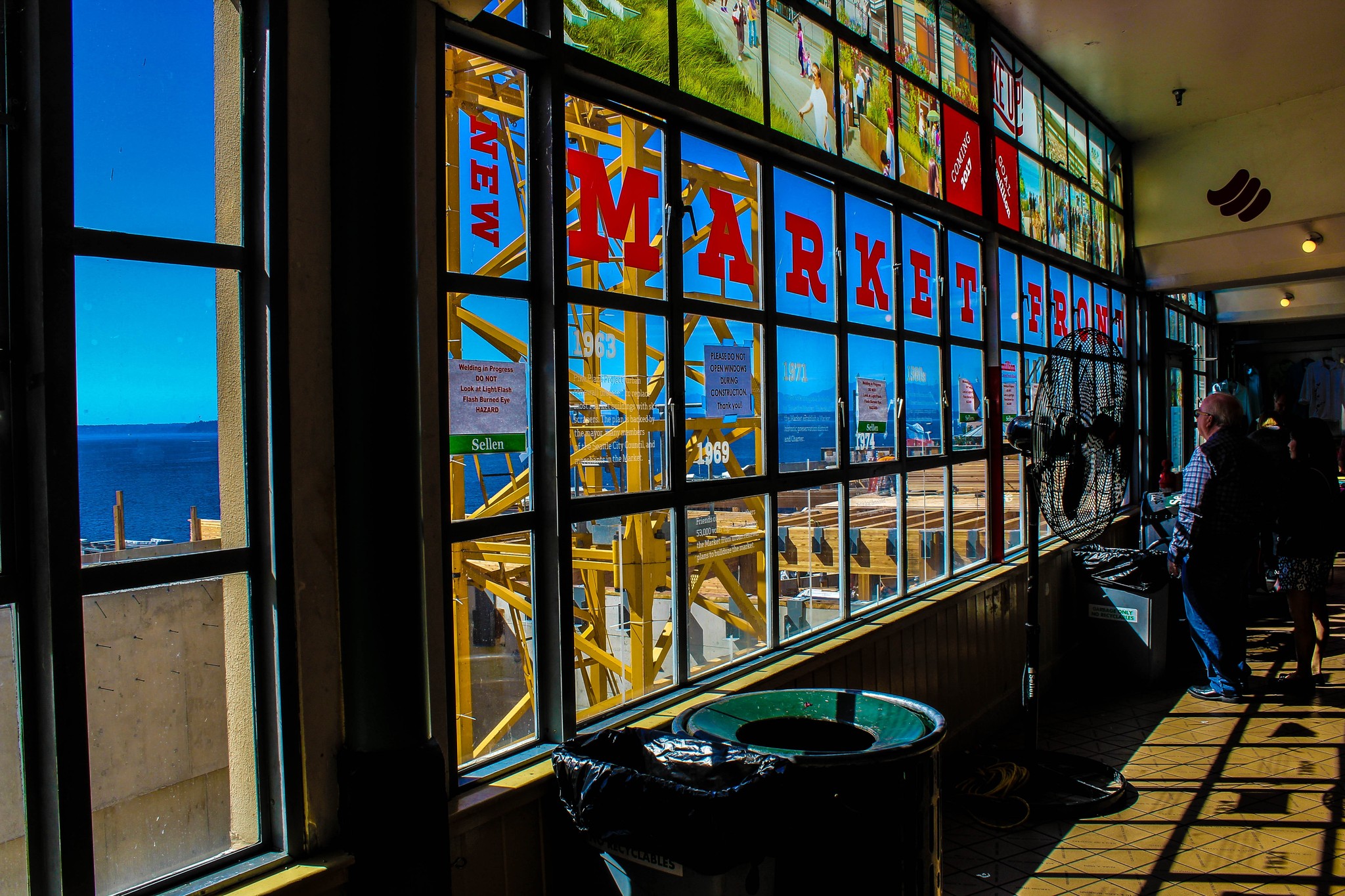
[1194,407,1215,422]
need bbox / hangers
[1323,350,1336,362]
[1226,377,1236,384]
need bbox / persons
[798,63,832,153]
[1029,191,1118,275]
[797,21,812,81]
[919,108,941,163]
[927,159,940,199]
[880,107,900,179]
[1248,386,1345,698]
[720,0,758,61]
[833,65,872,155]
[1167,393,1271,703]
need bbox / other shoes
[1278,672,1324,690]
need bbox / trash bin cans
[1073,547,1170,678]
[671,687,950,896]
[553,730,782,896]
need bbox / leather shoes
[1185,683,1244,704]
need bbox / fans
[955,327,1137,815]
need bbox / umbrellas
[927,110,939,124]
